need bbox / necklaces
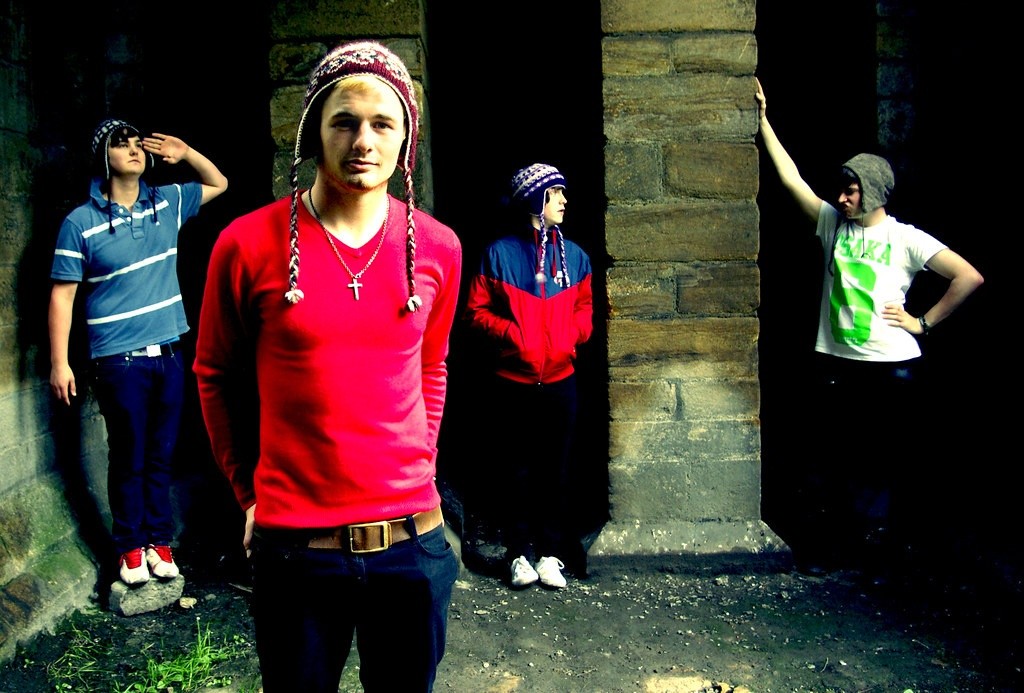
[309,185,389,300]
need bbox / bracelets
[919,316,930,333]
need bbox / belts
[300,505,444,553]
[117,342,178,357]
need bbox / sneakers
[145,544,179,578]
[510,556,538,586]
[120,546,149,584]
[535,554,566,587]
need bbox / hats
[285,40,422,312]
[827,153,895,277]
[512,163,570,288]
[92,120,160,235]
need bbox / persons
[48,121,229,583]
[754,76,984,573]
[468,164,594,588]
[194,41,461,693]
